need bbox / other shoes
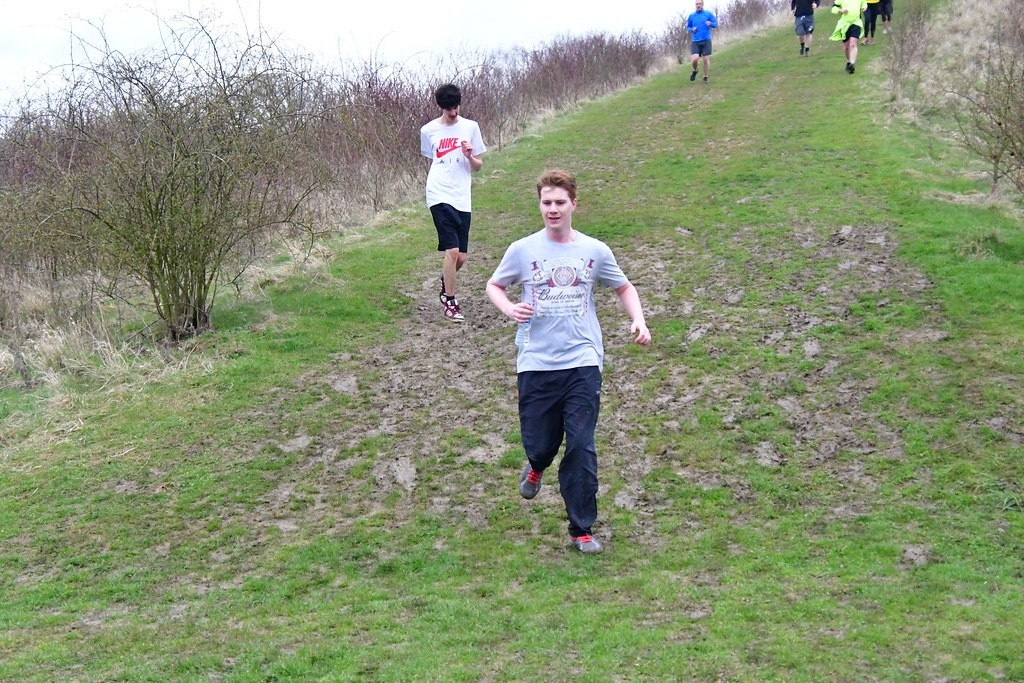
[800,48,804,56]
[805,51,811,57]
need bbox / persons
[484,168,654,555]
[860,1,880,46]
[877,0,894,34]
[790,0,821,56]
[684,0,719,84]
[418,85,487,325]
[830,0,868,76]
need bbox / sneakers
[846,61,850,71]
[703,77,709,83]
[848,64,856,74]
[861,38,868,45]
[870,38,875,44]
[690,70,698,81]
[571,535,602,554]
[444,298,465,323]
[439,276,448,305]
[518,463,545,500]
